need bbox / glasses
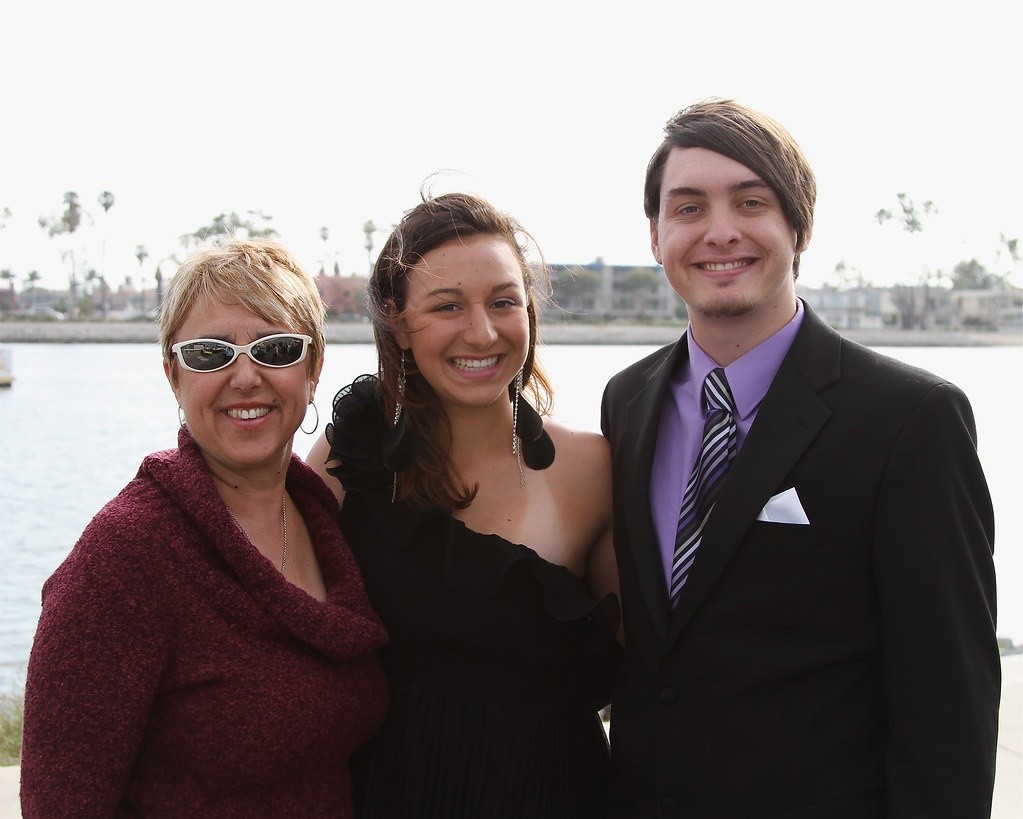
[171,333,314,373]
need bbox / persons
[601,98,1001,819]
[21,243,388,819]
[303,193,626,819]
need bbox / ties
[667,371,736,614]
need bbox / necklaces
[226,493,288,573]
